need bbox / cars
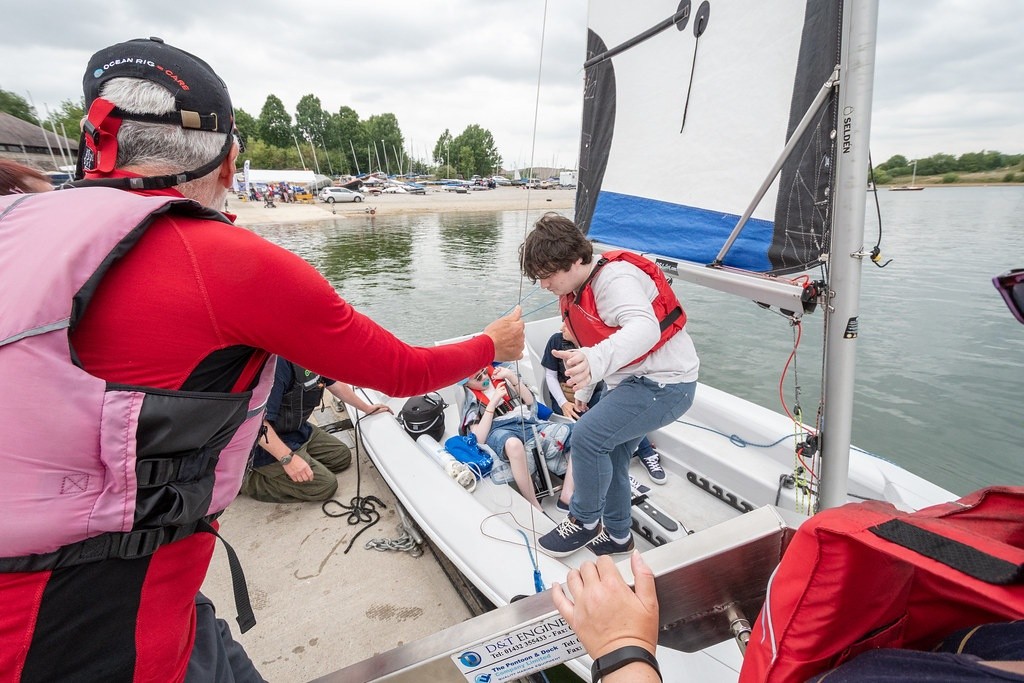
[318,186,365,204]
[291,133,577,196]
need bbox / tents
[233,169,319,200]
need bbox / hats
[83,37,240,135]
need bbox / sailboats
[340,0,991,683]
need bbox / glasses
[474,368,488,381]
[992,267,1024,325]
[425,391,443,405]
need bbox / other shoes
[556,495,571,513]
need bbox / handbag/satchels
[397,391,446,442]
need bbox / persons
[541,322,666,496]
[0,37,525,683]
[248,183,294,208]
[457,366,577,512]
[550,549,1024,683]
[518,210,701,558]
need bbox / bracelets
[591,645,664,683]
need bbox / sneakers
[537,511,603,558]
[585,527,635,556]
[640,454,667,485]
